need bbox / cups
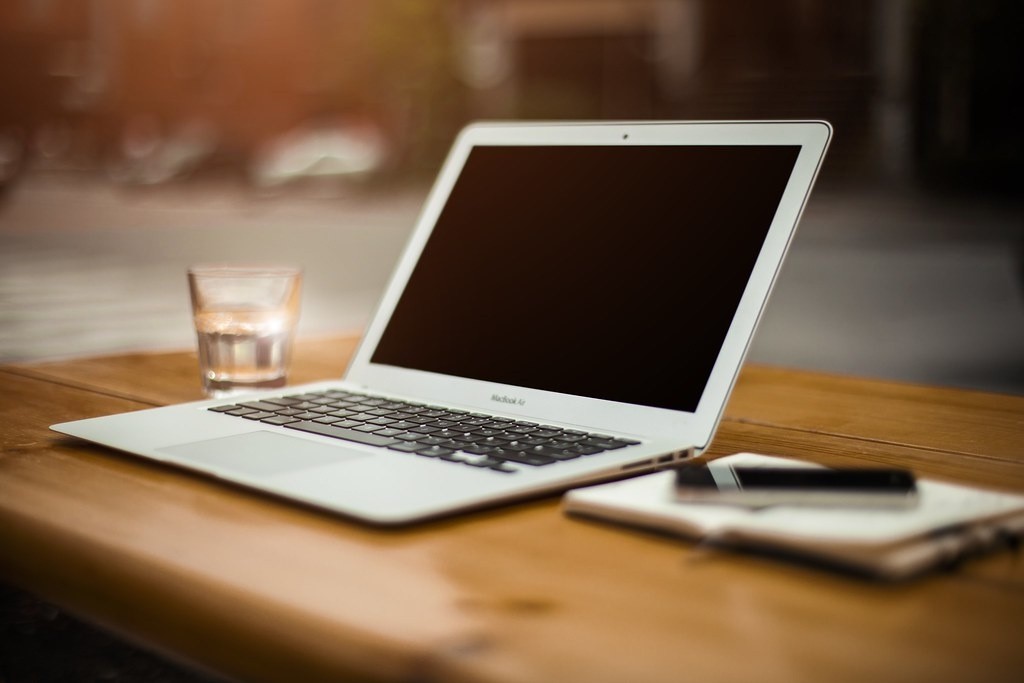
[188,270,300,399]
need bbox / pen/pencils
[674,463,915,495]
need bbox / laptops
[48,118,833,527]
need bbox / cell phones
[675,464,919,509]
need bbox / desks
[0,331,1024,683]
